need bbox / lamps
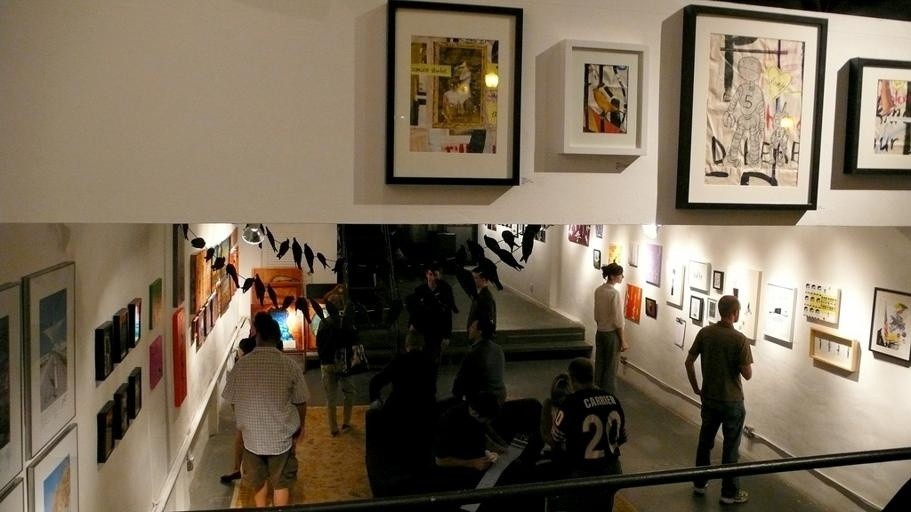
[242,223,266,245]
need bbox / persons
[543,357,628,511]
[685,297,752,505]
[454,261,507,405]
[593,263,628,394]
[315,293,355,436]
[220,338,268,484]
[422,384,509,491]
[248,314,269,336]
[225,319,308,508]
[365,332,439,410]
[406,266,457,367]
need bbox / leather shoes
[221,470,241,482]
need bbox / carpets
[230,404,637,512]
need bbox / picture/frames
[707,298,717,322]
[646,297,657,318]
[689,295,704,321]
[95,296,143,382]
[559,38,648,156]
[0,258,79,512]
[808,327,859,374]
[869,287,910,362]
[192,292,216,349]
[386,0,522,187]
[843,57,911,176]
[97,367,143,464]
[676,4,830,211]
[713,269,723,290]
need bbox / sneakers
[720,488,750,504]
[694,479,712,494]
[342,424,351,433]
[331,430,339,439]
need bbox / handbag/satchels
[334,340,376,377]
